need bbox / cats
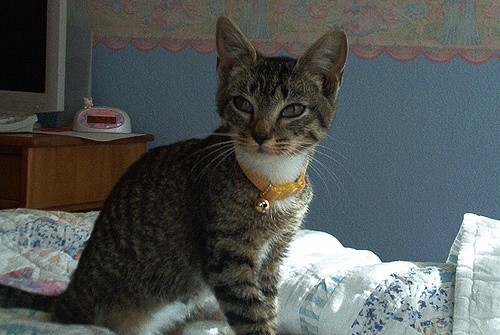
[0,16,348,335]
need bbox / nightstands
[0,133,155,212]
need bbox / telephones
[0,113,39,132]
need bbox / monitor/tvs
[1,1,65,114]
